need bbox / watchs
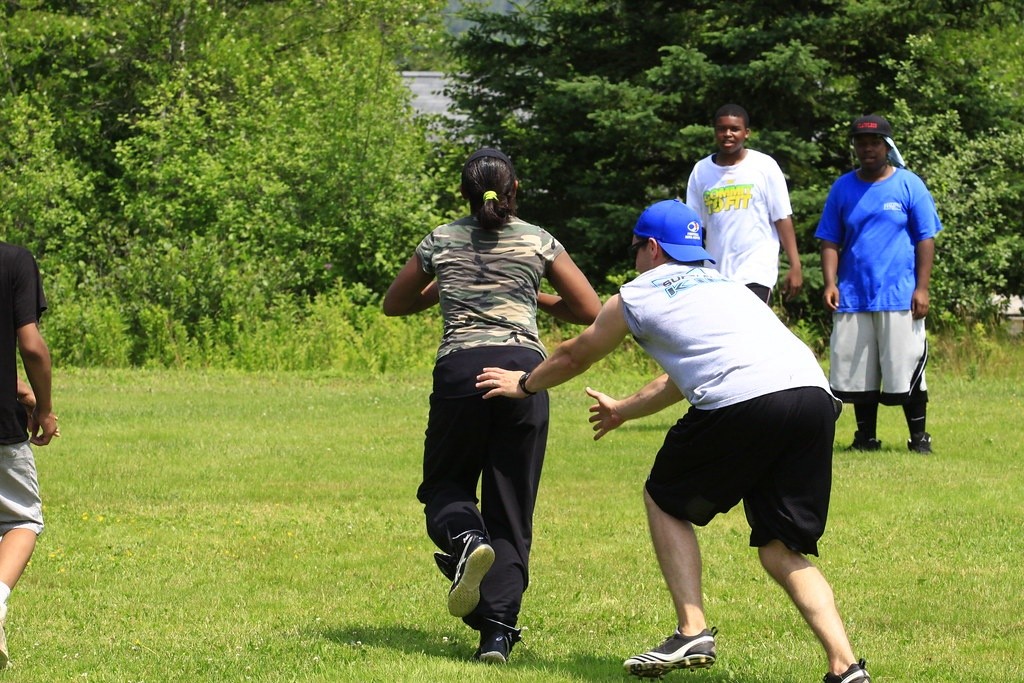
[519,373,537,395]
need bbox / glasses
[627,239,648,259]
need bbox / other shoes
[0,622,8,667]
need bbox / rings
[493,380,496,387]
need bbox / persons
[686,104,804,306]
[382,148,602,664]
[814,116,943,452]
[0,241,60,670]
[476,199,873,683]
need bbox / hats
[634,199,716,264]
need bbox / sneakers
[470,632,510,663]
[448,530,496,617]
[844,430,881,452]
[908,432,931,454]
[823,659,870,683]
[622,626,719,679]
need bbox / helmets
[848,116,893,138]
